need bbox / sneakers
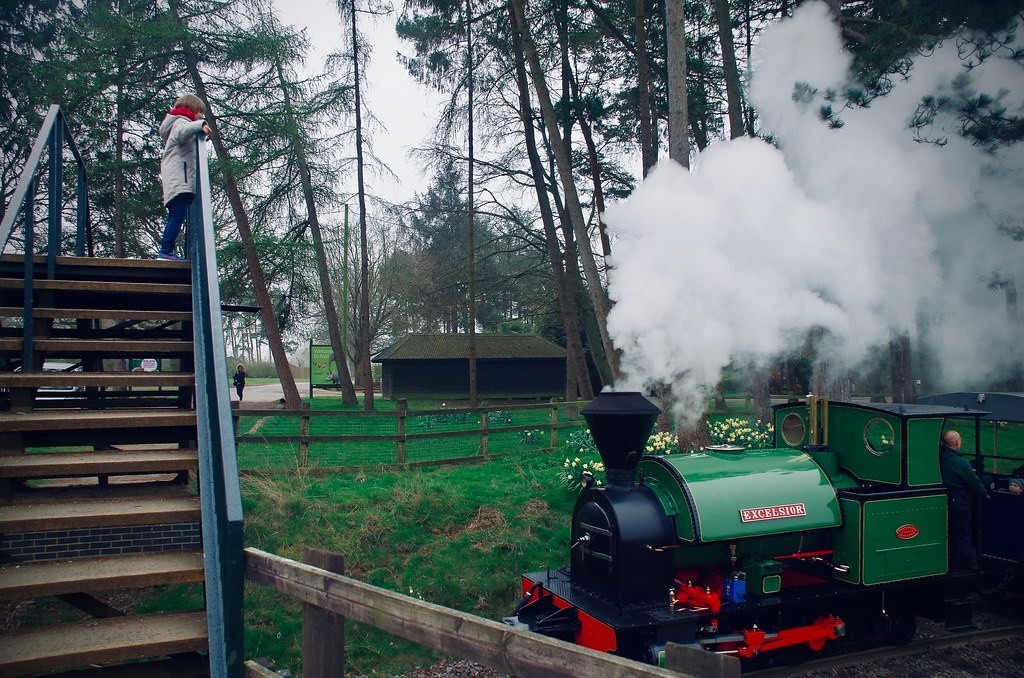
[156,252,186,261]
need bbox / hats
[1008,478,1023,490]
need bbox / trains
[496,392,1023,674]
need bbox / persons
[156,95,212,261]
[1009,478,1024,494]
[233,364,246,401]
[939,430,992,573]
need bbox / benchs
[997,477,1024,495]
